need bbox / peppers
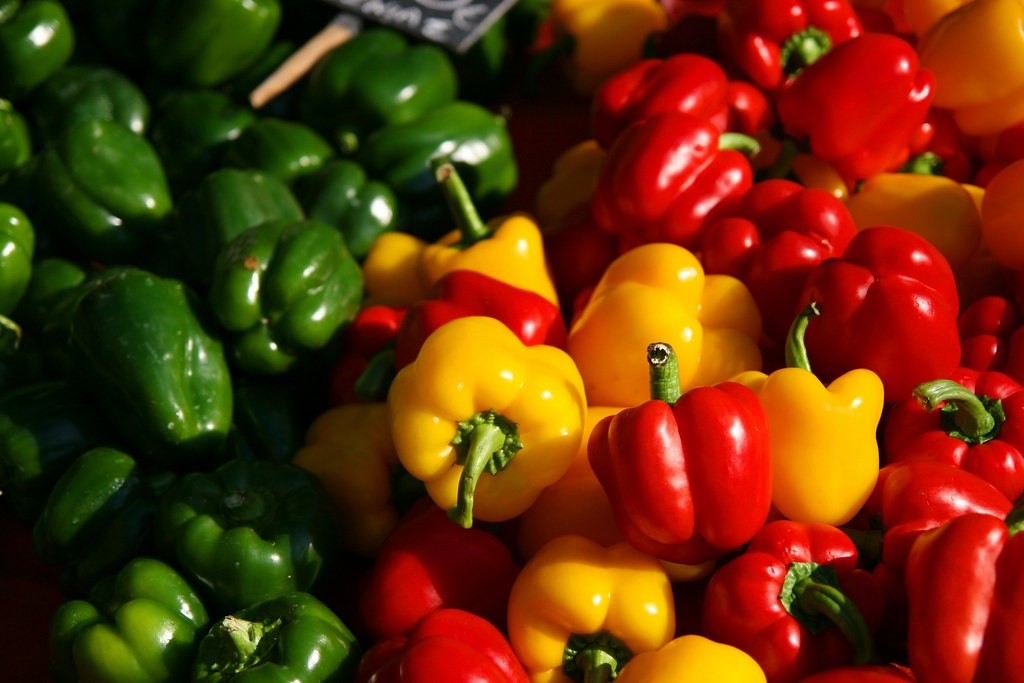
[0,0,1024,683]
